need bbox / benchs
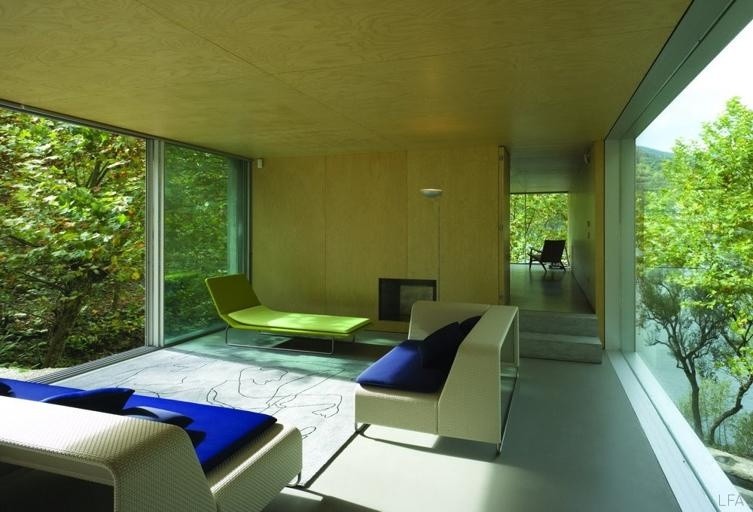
[355,300,520,456]
[1,379,303,511]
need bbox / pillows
[419,321,459,369]
[40,387,194,429]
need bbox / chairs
[204,272,372,356]
[527,240,566,273]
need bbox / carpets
[49,329,408,487]
